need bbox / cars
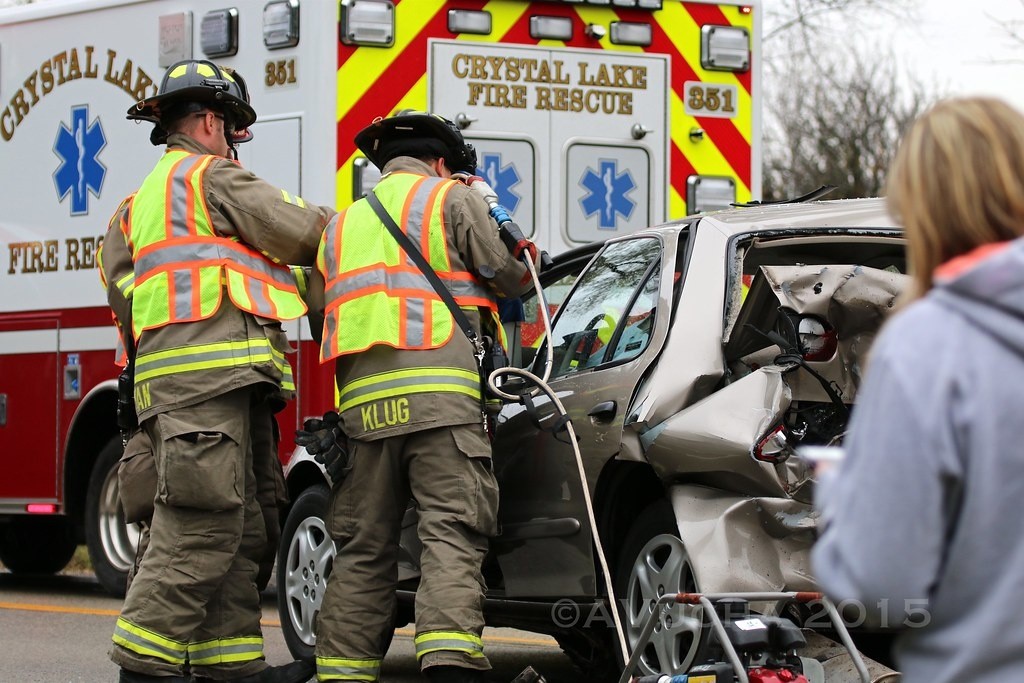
[278,198,912,677]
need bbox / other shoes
[120,656,316,683]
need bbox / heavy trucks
[1,1,763,596]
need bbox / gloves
[294,411,347,483]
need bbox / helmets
[353,109,468,171]
[125,60,257,145]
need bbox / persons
[97,65,288,595]
[797,94,1023,683]
[306,112,527,682]
[95,56,336,682]
